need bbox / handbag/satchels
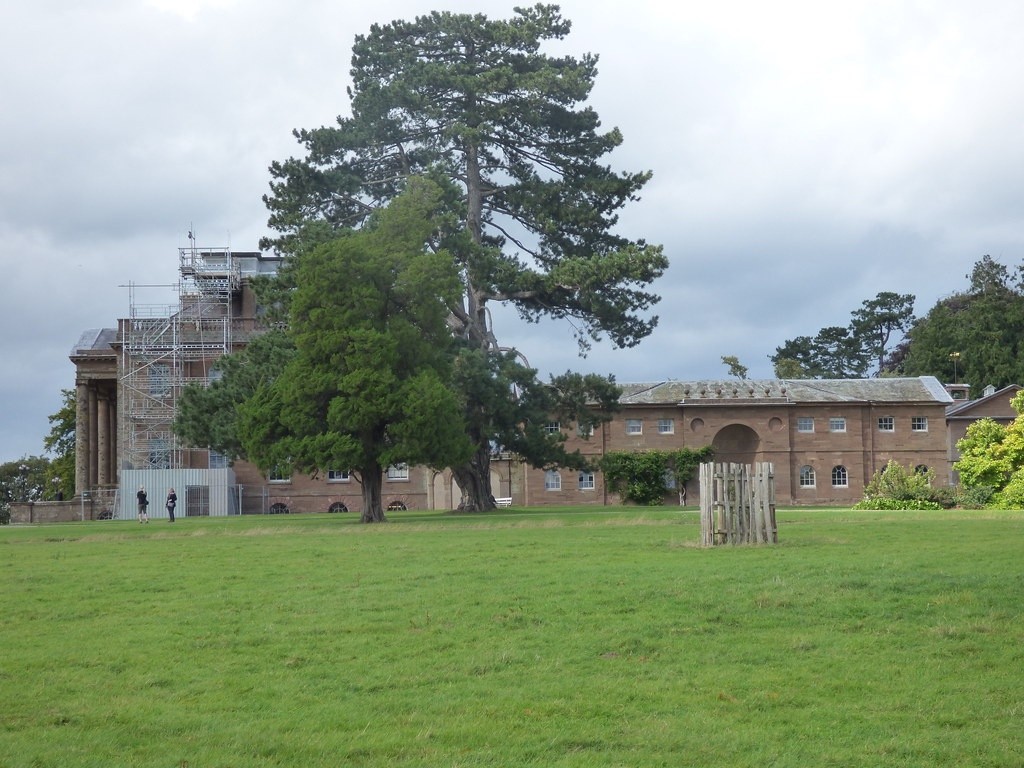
[168,502,174,508]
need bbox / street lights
[19,464,30,501]
[4,503,10,513]
[52,477,61,500]
[950,351,961,381]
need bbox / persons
[137,486,148,523]
[166,488,177,522]
[57,489,63,501]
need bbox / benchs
[495,497,512,508]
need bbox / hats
[169,488,175,492]
[139,485,144,489]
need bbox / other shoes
[138,521,142,524]
[168,520,174,522]
[145,520,148,524]
